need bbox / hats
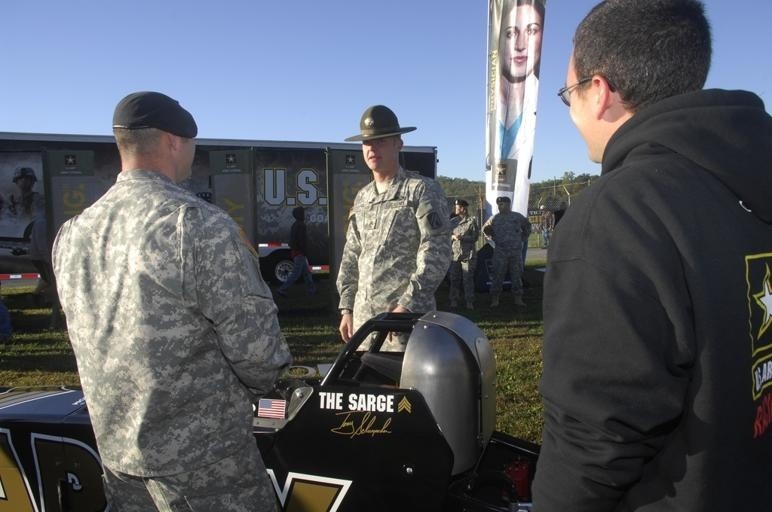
[113,92,197,138]
[497,197,510,204]
[454,199,468,207]
[344,106,417,141]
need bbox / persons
[8,166,41,212]
[449,197,479,310]
[334,105,454,352]
[487,1,546,197]
[276,206,319,298]
[484,196,533,309]
[527,0,771,510]
[536,202,555,252]
[50,91,296,511]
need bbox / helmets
[13,168,37,183]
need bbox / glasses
[557,76,617,106]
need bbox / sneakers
[450,300,457,307]
[467,302,474,311]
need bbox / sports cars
[1,301,542,512]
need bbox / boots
[515,295,527,307]
[490,296,499,308]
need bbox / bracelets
[339,309,351,316]
